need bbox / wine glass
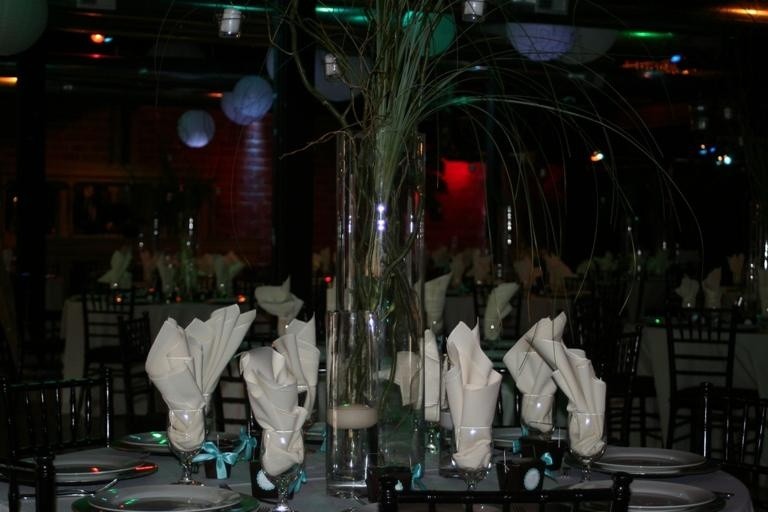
[259,431,306,511]
[167,391,214,484]
[452,421,491,488]
[566,411,605,485]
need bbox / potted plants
[153,0,708,480]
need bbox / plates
[492,426,569,448]
[89,483,241,512]
[585,445,709,475]
[568,478,716,511]
[304,421,328,441]
[50,453,143,483]
[122,429,240,453]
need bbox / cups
[436,351,494,479]
[523,395,559,439]
[249,458,295,503]
[366,466,411,502]
[200,438,235,479]
[326,309,376,500]
[497,457,546,492]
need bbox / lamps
[217,8,242,42]
[461,1,487,24]
[322,50,343,81]
